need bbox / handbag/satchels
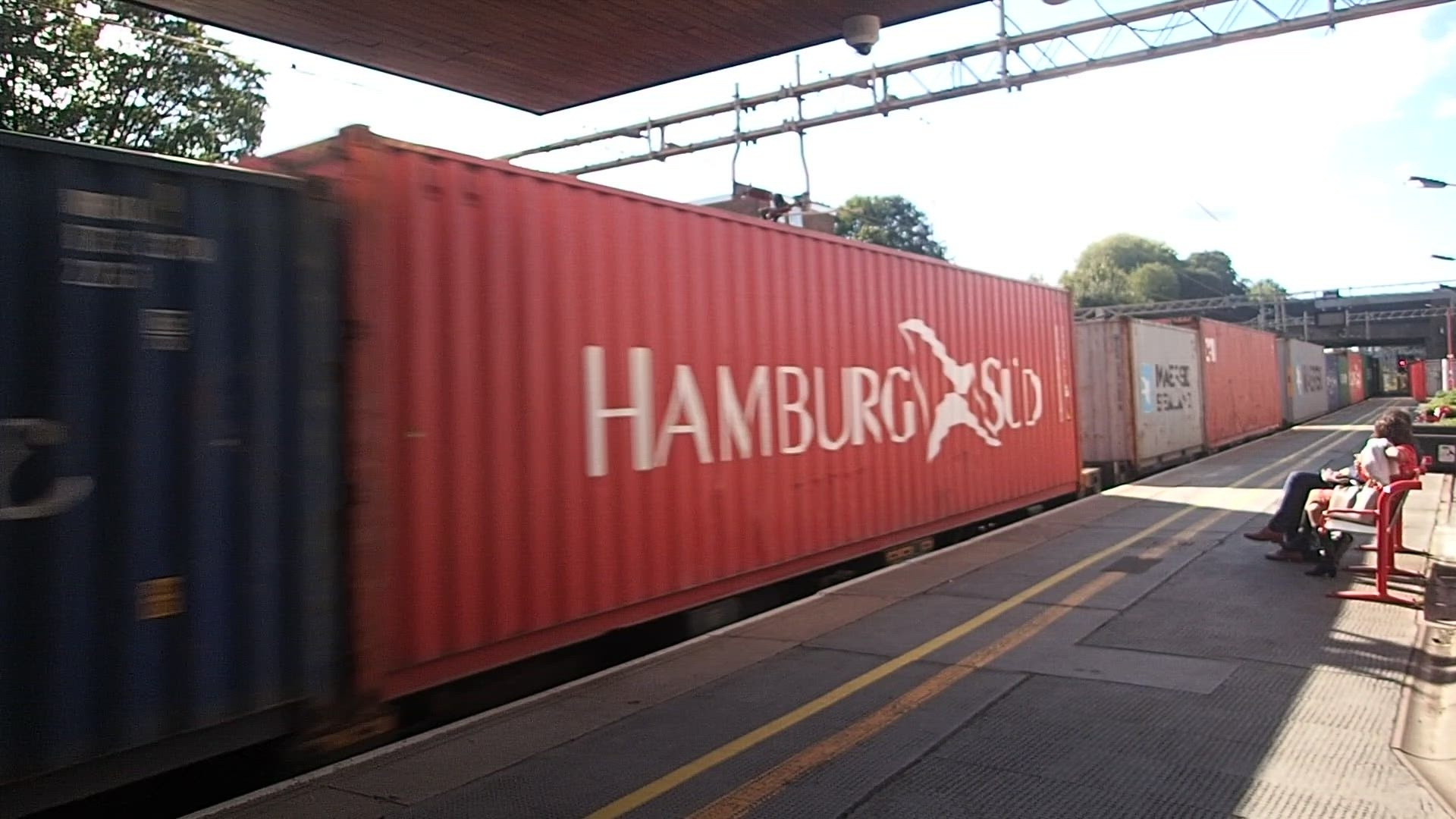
[1327,477,1378,525]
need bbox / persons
[1243,406,1432,581]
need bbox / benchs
[1322,461,1427,610]
[1416,405,1452,422]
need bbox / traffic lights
[1398,356,1407,373]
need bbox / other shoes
[1243,525,1284,544]
[1266,547,1305,562]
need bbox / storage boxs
[228,123,1083,698]
[1337,353,1350,408]
[1073,315,1205,473]
[0,124,352,819]
[1156,316,1283,451]
[1275,338,1330,425]
[1324,352,1341,412]
[1362,354,1373,399]
[1371,357,1385,395]
[1346,351,1365,405]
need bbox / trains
[0,122,1385,819]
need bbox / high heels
[1306,560,1338,577]
